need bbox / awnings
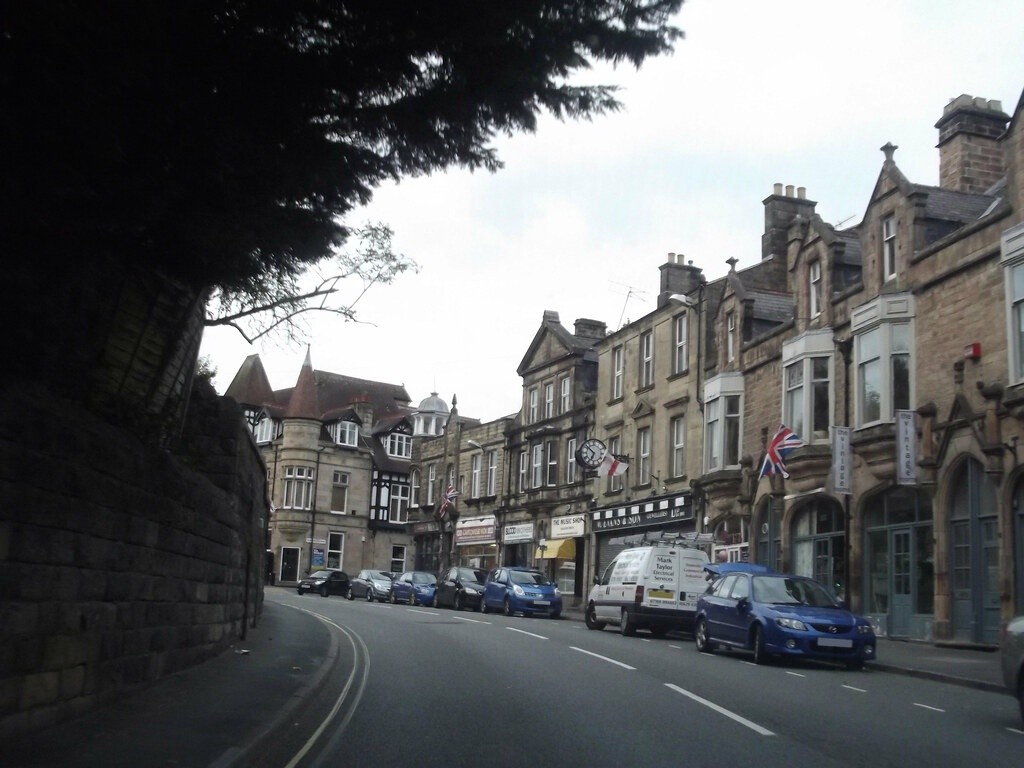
[535,538,576,559]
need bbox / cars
[432,567,489,611]
[345,569,395,603]
[480,567,562,620]
[389,571,438,606]
[296,569,350,598]
[1002,616,1024,724]
[693,562,877,665]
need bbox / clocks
[574,438,608,469]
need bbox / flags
[598,452,629,476]
[757,424,802,482]
[439,484,460,518]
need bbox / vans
[584,546,715,637]
